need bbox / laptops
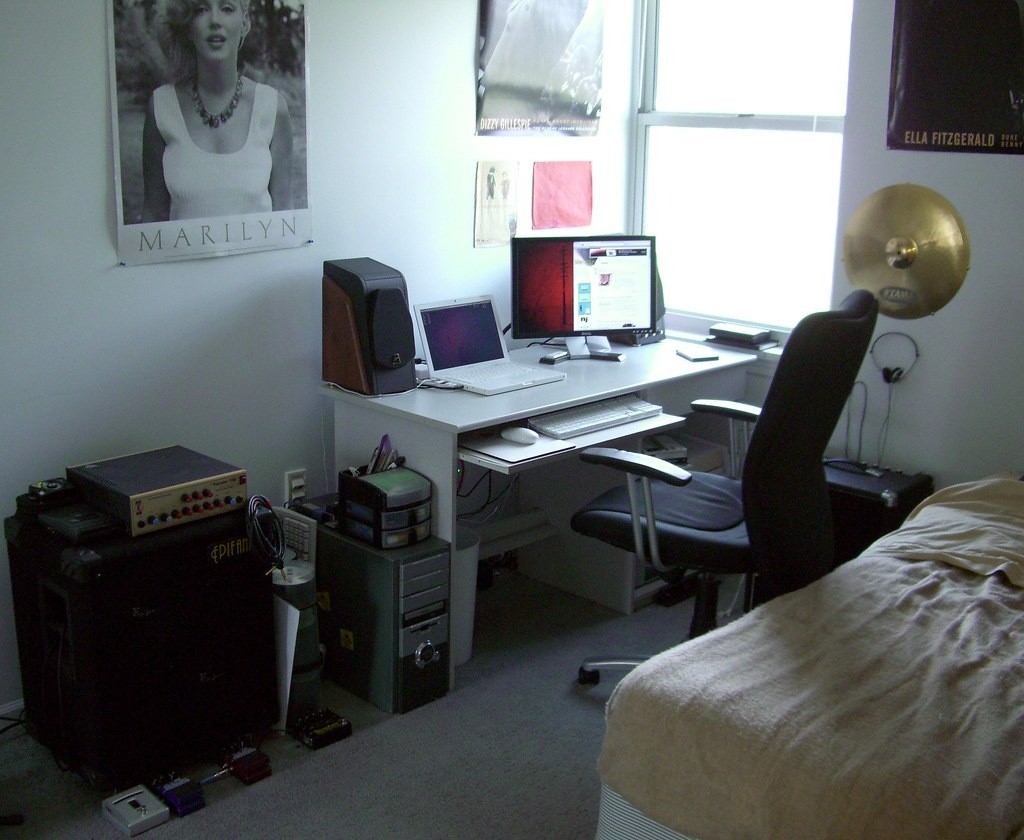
[413,294,567,395]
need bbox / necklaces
[192,72,243,128]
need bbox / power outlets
[284,468,307,509]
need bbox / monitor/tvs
[510,236,657,365]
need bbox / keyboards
[527,392,663,440]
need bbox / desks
[313,320,757,695]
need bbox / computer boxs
[292,492,453,713]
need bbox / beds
[594,471,1024,840]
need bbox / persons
[143,0,295,220]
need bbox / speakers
[607,269,666,346]
[3,502,282,798]
[741,456,933,618]
[321,257,416,396]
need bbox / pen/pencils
[366,434,404,474]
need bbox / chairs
[569,290,881,686]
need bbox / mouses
[500,426,539,445]
